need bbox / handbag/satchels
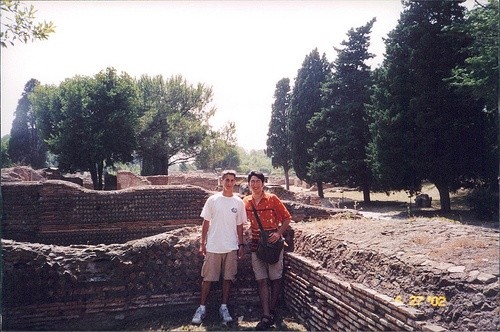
[256,231,282,265]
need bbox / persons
[242,170,292,331]
[191,169,247,325]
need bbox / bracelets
[239,244,244,247]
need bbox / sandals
[256,315,274,329]
[274,313,287,329]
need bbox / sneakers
[219,308,232,322]
[191,307,206,324]
